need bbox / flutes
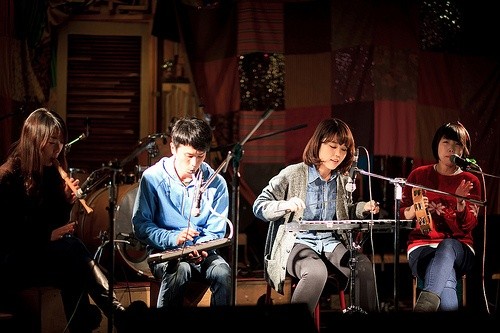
[51,155,94,215]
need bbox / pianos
[147,237,234,263]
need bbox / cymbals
[411,186,432,234]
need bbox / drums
[71,181,155,277]
[68,164,92,200]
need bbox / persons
[399,120,482,313]
[252,118,380,314]
[0,107,148,333]
[131,116,236,308]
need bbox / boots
[59,274,102,333]
[413,291,440,315]
[78,260,148,333]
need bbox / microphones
[450,154,476,169]
[346,148,359,192]
[75,171,95,199]
[191,170,203,218]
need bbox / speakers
[115,302,317,333]
[318,308,500,333]
